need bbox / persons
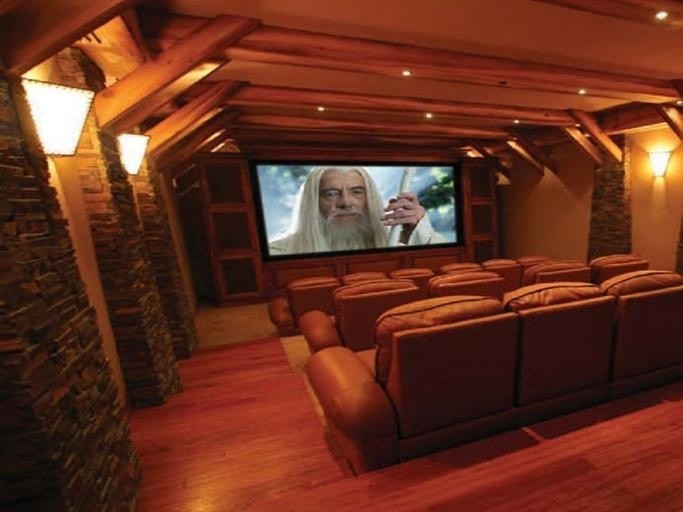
[269,166,447,256]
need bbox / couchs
[268,255,550,335]
[306,272,682,472]
[299,257,651,358]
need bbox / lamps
[21,78,97,156]
[117,133,150,175]
[646,150,673,176]
